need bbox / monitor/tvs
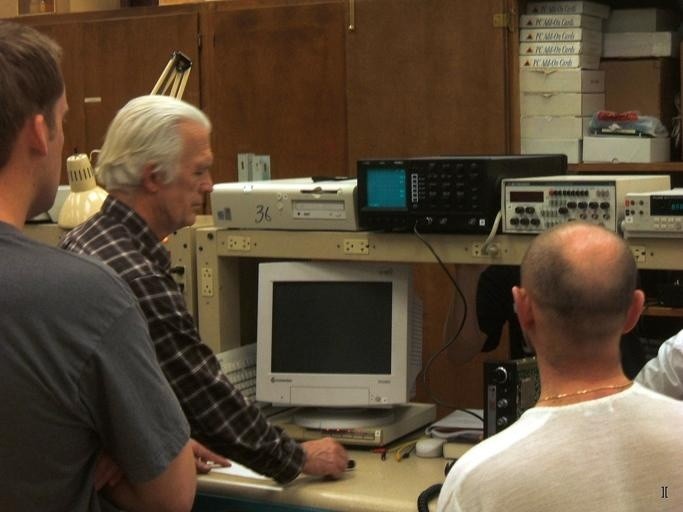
[256,261,435,427]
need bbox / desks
[195,435,478,512]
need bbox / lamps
[58,152,108,230]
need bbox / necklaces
[537,381,636,405]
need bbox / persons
[0,20,198,511]
[436,220,682,512]
[632,327,682,399]
[58,93,350,484]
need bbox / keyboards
[211,343,268,413]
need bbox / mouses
[415,432,447,458]
